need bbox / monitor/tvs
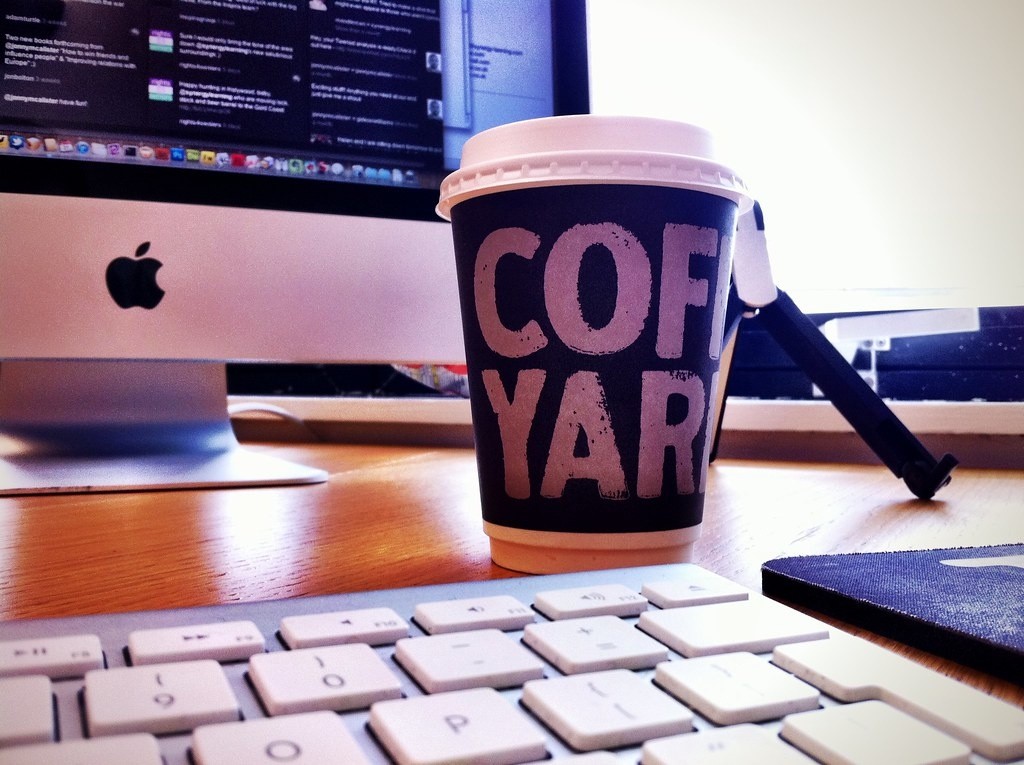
[0,0,589,495]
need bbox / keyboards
[0,563,1024,765]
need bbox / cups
[435,114,753,571]
